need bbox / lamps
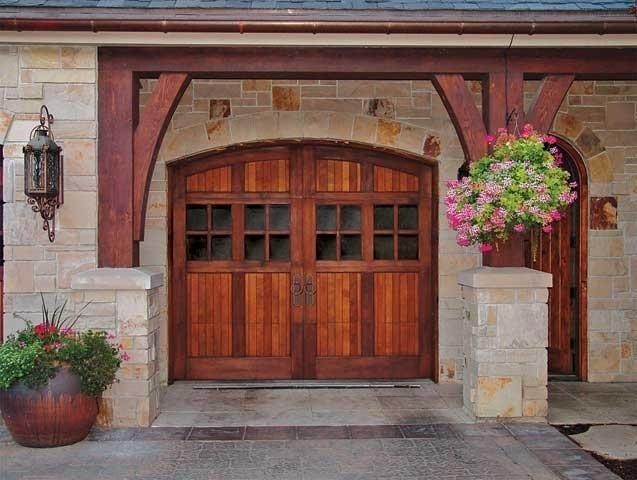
[20,105,63,242]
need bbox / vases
[0,359,100,447]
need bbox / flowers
[445,123,576,252]
[0,298,129,386]
